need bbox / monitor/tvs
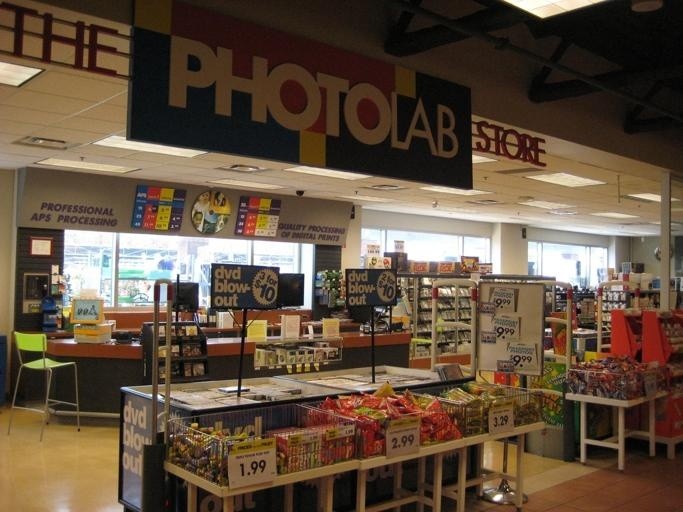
[172,282,198,312]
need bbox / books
[151,324,205,378]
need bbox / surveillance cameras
[296,190,305,197]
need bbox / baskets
[568,361,670,401]
[401,380,544,438]
[299,389,466,462]
[162,402,359,487]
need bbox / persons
[191,211,203,228]
[194,191,209,208]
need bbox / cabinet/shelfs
[395,256,682,460]
[144,322,212,386]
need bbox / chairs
[7,330,81,442]
